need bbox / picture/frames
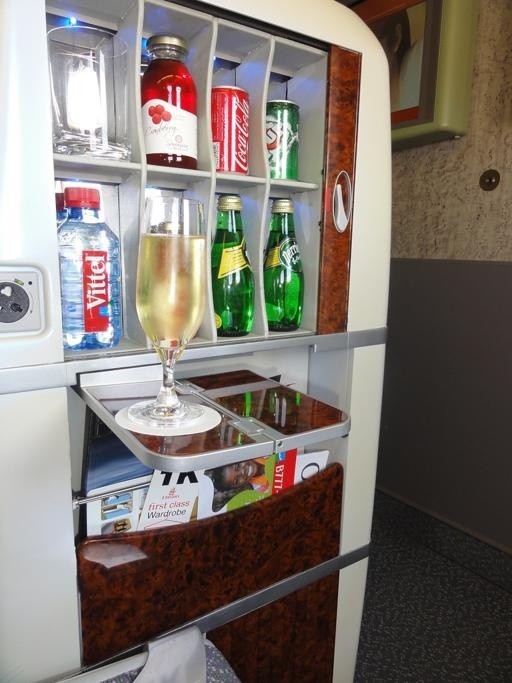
[350,0,480,152]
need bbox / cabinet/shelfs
[2,0,391,678]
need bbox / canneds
[209,85,249,175]
[264,100,299,180]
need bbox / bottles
[56,187,122,351]
[211,195,253,335]
[140,35,198,167]
[262,198,304,331]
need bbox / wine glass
[129,196,209,424]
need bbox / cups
[48,25,129,159]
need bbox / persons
[211,457,270,493]
